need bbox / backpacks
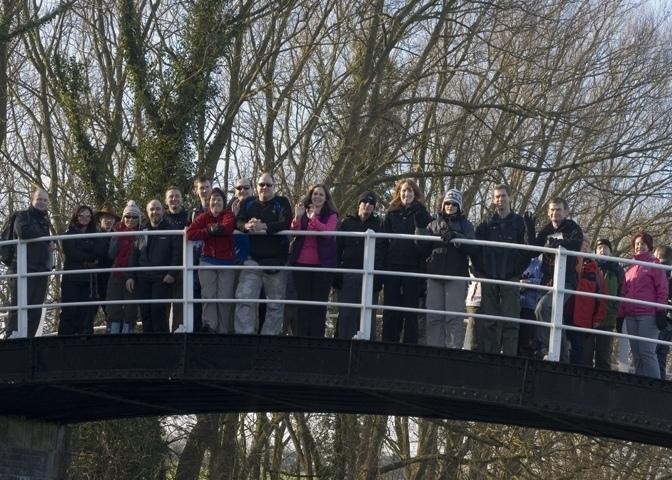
[0,211,27,267]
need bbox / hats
[358,192,376,205]
[595,239,613,253]
[122,201,140,218]
[441,189,462,206]
[95,204,121,220]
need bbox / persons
[591,237,626,371]
[470,185,531,357]
[653,244,672,379]
[616,232,669,380]
[226,178,257,266]
[563,232,607,369]
[58,205,105,336]
[523,197,583,364]
[185,173,212,331]
[163,185,188,230]
[187,187,236,333]
[125,200,182,333]
[108,200,147,334]
[291,184,338,338]
[413,188,479,349]
[518,257,541,356]
[336,190,384,339]
[0,188,57,337]
[384,178,432,344]
[235,172,292,334]
[90,202,121,335]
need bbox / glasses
[236,186,250,190]
[258,183,274,187]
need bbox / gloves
[208,224,224,236]
[548,238,563,248]
[414,213,426,229]
[441,232,456,240]
[523,212,535,229]
